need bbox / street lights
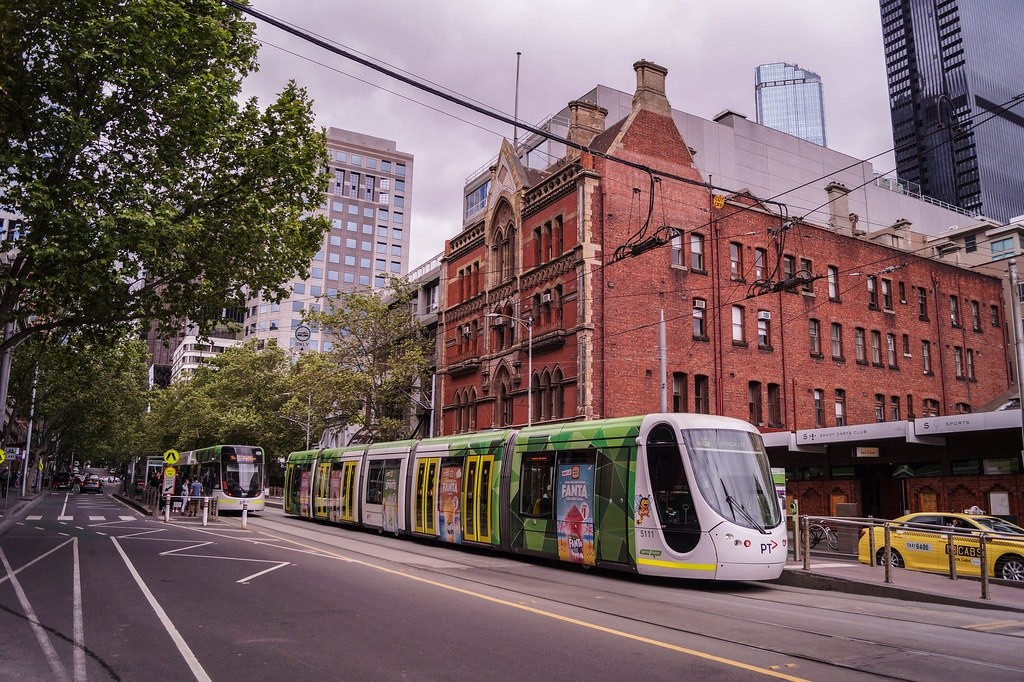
[487,312,532,426]
[279,414,309,451]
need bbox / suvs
[54,472,81,490]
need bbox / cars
[858,505,1024,581]
[80,478,103,493]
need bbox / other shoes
[181,512,187,516]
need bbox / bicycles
[799,518,840,550]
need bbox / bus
[163,445,265,515]
[125,456,164,498]
[284,412,788,581]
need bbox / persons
[11,470,24,486]
[150,473,189,516]
[73,476,81,496]
[188,474,204,517]
[539,483,552,516]
[51,474,59,491]
[0,467,12,498]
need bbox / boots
[188,511,192,516]
[194,509,197,516]
